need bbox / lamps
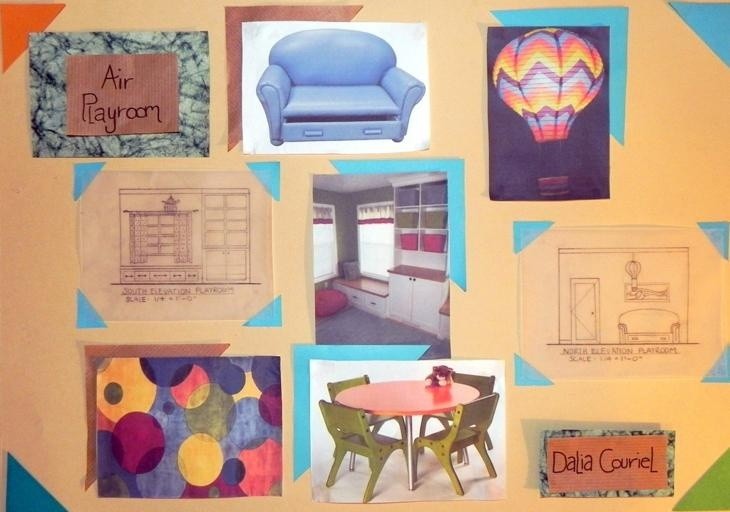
[318,372,499,504]
[256,27,426,148]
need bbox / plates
[350,289,388,319]
[388,273,449,340]
[393,178,449,254]
